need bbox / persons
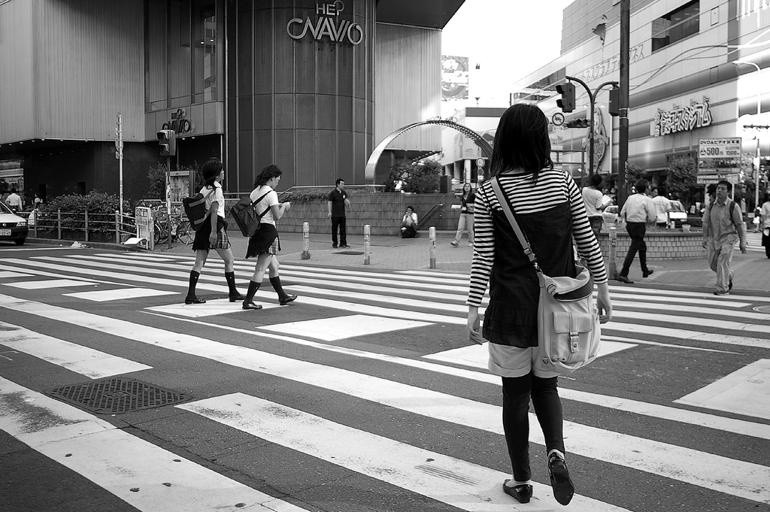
[701,180,750,295]
[32,191,44,204]
[401,205,418,238]
[759,190,770,259]
[582,174,672,284]
[326,178,351,248]
[185,157,245,304]
[242,164,298,309]
[4,187,22,210]
[466,104,612,505]
[752,204,761,233]
[450,183,475,247]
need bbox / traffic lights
[567,118,589,129]
[554,82,575,113]
[156,130,176,159]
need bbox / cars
[668,200,691,227]
[602,206,618,224]
[0,199,29,246]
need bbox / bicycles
[141,202,196,245]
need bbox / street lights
[731,60,762,210]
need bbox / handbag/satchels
[230,197,261,237]
[183,192,208,231]
[537,264,601,375]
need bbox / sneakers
[729,269,734,290]
[713,290,729,295]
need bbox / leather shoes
[185,298,206,304]
[547,452,574,506]
[503,479,533,503]
[617,276,633,284]
[229,295,246,302]
[643,270,653,277]
[243,302,262,309]
[279,294,297,305]
